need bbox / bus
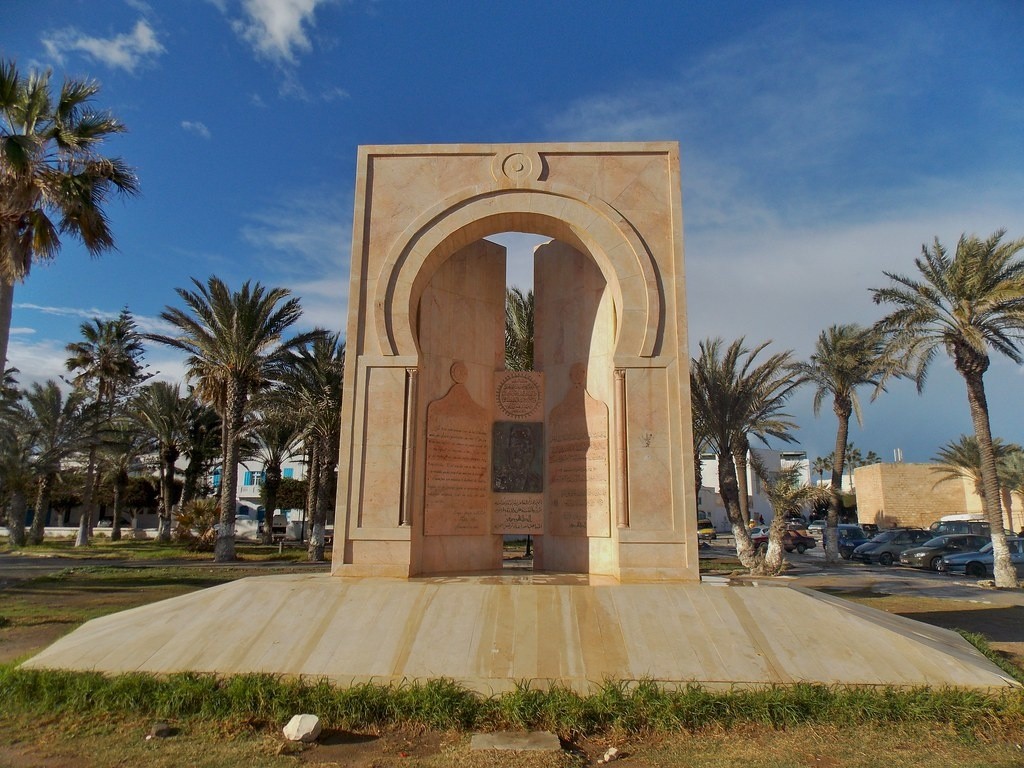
[930,514,991,538]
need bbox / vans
[822,524,871,559]
[698,519,717,540]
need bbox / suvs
[853,527,938,566]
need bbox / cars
[899,535,1019,571]
[808,520,827,534]
[751,530,817,554]
[938,538,1024,578]
[751,527,771,537]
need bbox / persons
[749,514,766,529]
[801,511,821,525]
[842,516,848,524]
